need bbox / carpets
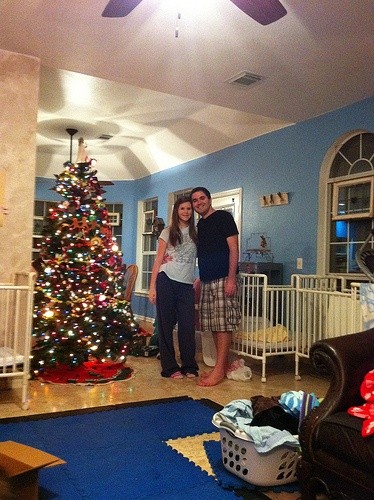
[40,360,137,386]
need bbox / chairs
[124,265,138,319]
[296,326,374,500]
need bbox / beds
[228,273,361,383]
[0,271,38,408]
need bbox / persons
[149,196,200,379]
[159,187,239,387]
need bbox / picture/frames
[142,210,155,234]
[108,213,119,226]
[333,176,374,220]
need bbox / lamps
[57,129,78,198]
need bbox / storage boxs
[0,440,67,500]
